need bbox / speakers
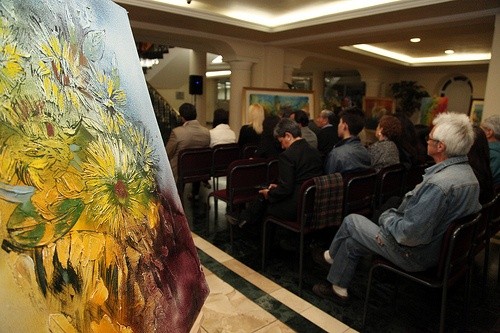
[188,74,203,95]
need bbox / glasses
[425,136,442,145]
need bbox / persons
[229,103,272,164]
[224,117,322,233]
[242,104,433,180]
[200,107,236,190]
[387,111,500,208]
[165,101,211,201]
[312,110,481,307]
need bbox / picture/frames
[418,97,448,128]
[241,87,318,126]
[0,0,210,333]
[467,98,484,128]
[362,96,396,122]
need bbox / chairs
[175,142,500,333]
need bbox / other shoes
[312,282,350,306]
[188,194,200,201]
[226,212,248,229]
[203,183,211,188]
[310,248,332,271]
